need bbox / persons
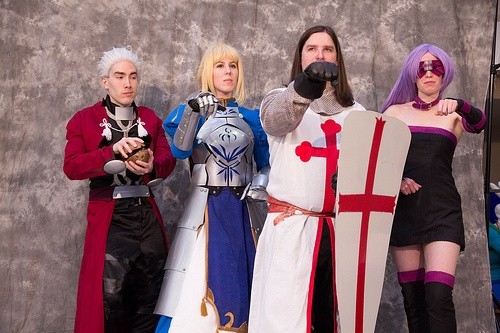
[247,26,365,333]
[63,48,176,333]
[380,43,490,333]
[155,44,270,333]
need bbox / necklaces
[412,96,441,110]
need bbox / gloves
[193,90,226,118]
[304,62,339,86]
